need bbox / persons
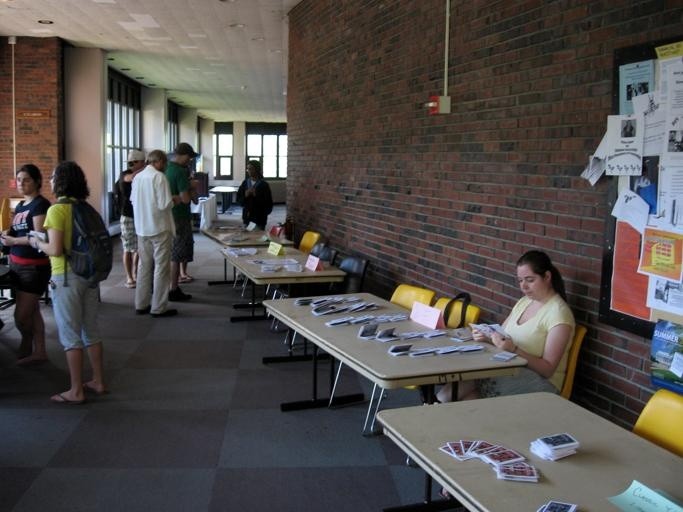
[436,250,576,403]
[118,143,201,318]
[27,161,105,402]
[236,160,273,231]
[0,163,52,365]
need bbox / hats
[127,148,143,161]
[173,142,199,156]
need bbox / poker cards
[529,433,580,461]
[537,500,577,512]
[438,440,540,483]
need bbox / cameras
[190,194,201,205]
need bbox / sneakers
[169,288,192,302]
[135,304,151,314]
[153,308,178,318]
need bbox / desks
[190,192,217,233]
[0,234,55,332]
[220,246,347,331]
[208,185,239,215]
[375,391,683,512]
[199,226,296,290]
[260,291,528,433]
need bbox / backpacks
[53,198,114,285]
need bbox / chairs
[318,247,338,266]
[298,231,321,254]
[558,323,588,400]
[330,256,369,294]
[402,297,481,403]
[270,224,286,239]
[629,388,683,459]
[311,239,329,257]
[389,283,433,313]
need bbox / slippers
[50,394,84,404]
[124,280,135,288]
[81,383,109,396]
[178,276,192,283]
